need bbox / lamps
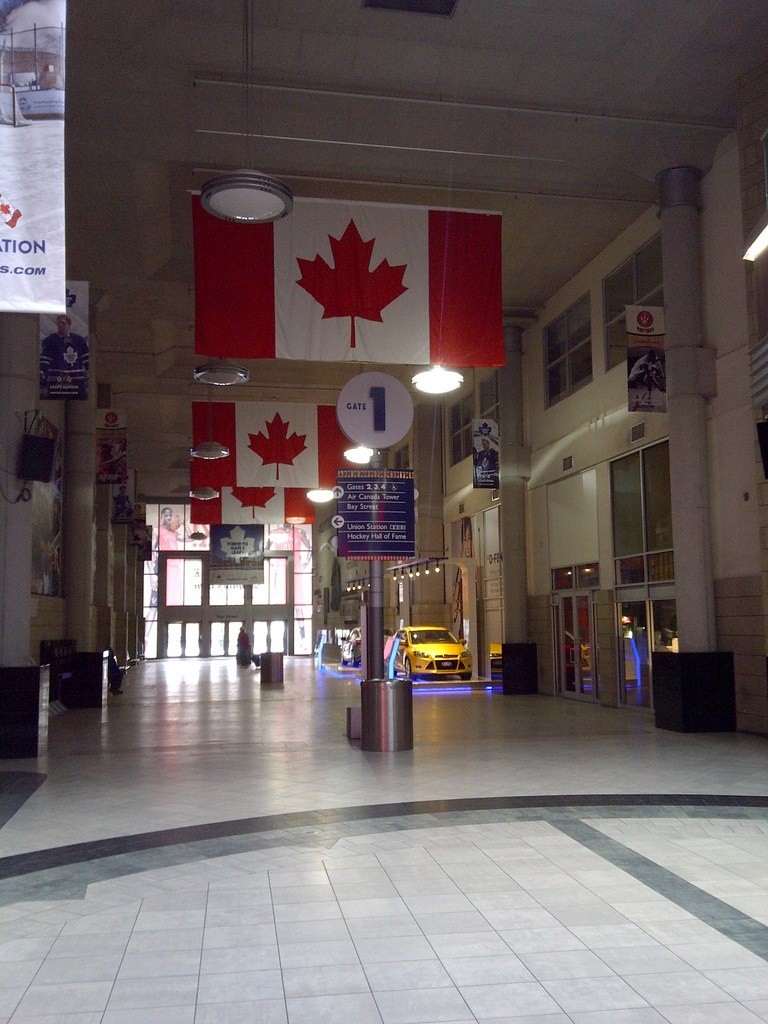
[401,568,404,579]
[352,583,356,590]
[343,446,374,465]
[358,581,361,589]
[190,532,206,540]
[425,563,429,575]
[435,560,440,572]
[392,571,397,581]
[411,367,465,395]
[409,567,413,578]
[189,486,219,500]
[307,489,335,503]
[416,564,421,576]
[193,357,251,387]
[199,0,296,224]
[346,584,351,591]
[189,384,230,459]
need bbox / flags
[190,487,319,526]
[189,398,364,488]
[188,187,508,367]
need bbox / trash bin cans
[260,652,283,683]
[359,677,413,751]
[0,665,51,757]
[75,648,110,706]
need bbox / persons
[475,438,499,479]
[114,484,131,513]
[460,517,473,558]
[145,508,195,636]
[40,316,90,380]
[237,625,253,666]
[267,525,312,638]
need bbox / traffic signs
[335,469,417,560]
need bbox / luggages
[250,655,262,670]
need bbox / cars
[566,632,595,663]
[488,641,505,670]
[390,626,473,682]
[339,626,363,667]
